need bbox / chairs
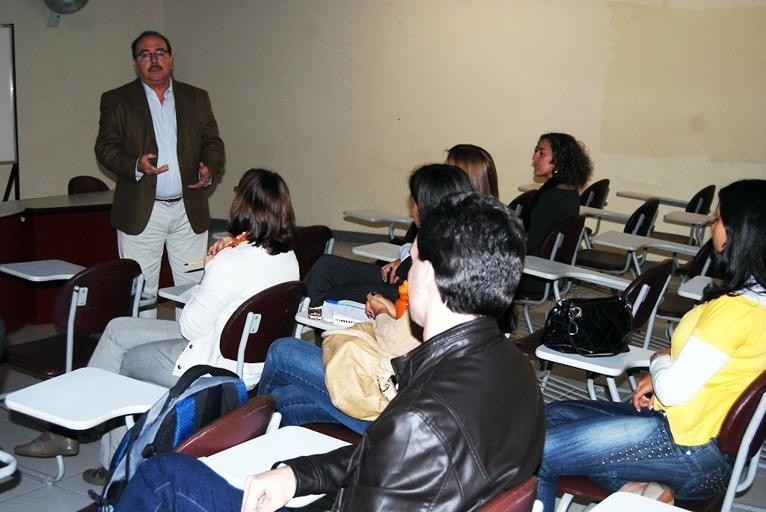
[344,207,414,240]
[195,424,539,511]
[518,178,610,238]
[594,231,718,341]
[295,306,347,331]
[158,280,311,381]
[354,241,403,261]
[665,211,715,247]
[616,184,716,248]
[575,198,659,280]
[595,230,718,281]
[524,254,678,350]
[6,366,283,457]
[678,275,724,304]
[0,257,145,483]
[502,215,586,341]
[536,341,766,512]
[213,224,336,281]
[67,175,110,194]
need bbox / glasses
[231,184,241,195]
[134,47,172,58]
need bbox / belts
[154,196,183,204]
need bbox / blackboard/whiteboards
[3,23,24,169]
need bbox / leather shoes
[13,432,81,460]
[83,466,118,484]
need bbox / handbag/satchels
[321,321,400,424]
[540,294,638,357]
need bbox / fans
[44,0,90,28]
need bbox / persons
[139,192,543,512]
[305,165,518,347]
[12,167,300,486]
[95,32,225,320]
[402,144,498,245]
[498,133,592,333]
[534,179,765,511]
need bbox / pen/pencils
[184,260,204,266]
[184,266,208,273]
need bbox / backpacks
[87,366,250,512]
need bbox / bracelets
[646,352,666,365]
